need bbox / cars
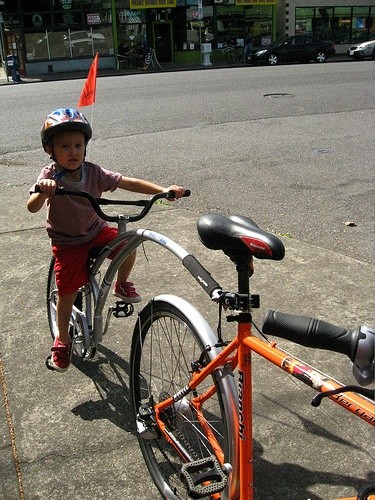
[252,34,336,65]
[243,34,272,62]
[347,39,375,61]
[65,32,106,49]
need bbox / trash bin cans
[201,43,212,66]
[7,54,22,82]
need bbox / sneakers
[51,335,71,373]
[113,281,141,303]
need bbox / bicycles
[226,46,244,64]
[33,184,375,500]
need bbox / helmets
[40,108,93,156]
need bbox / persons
[243,31,255,64]
[119,43,147,67]
[222,26,250,47]
[27,109,186,373]
